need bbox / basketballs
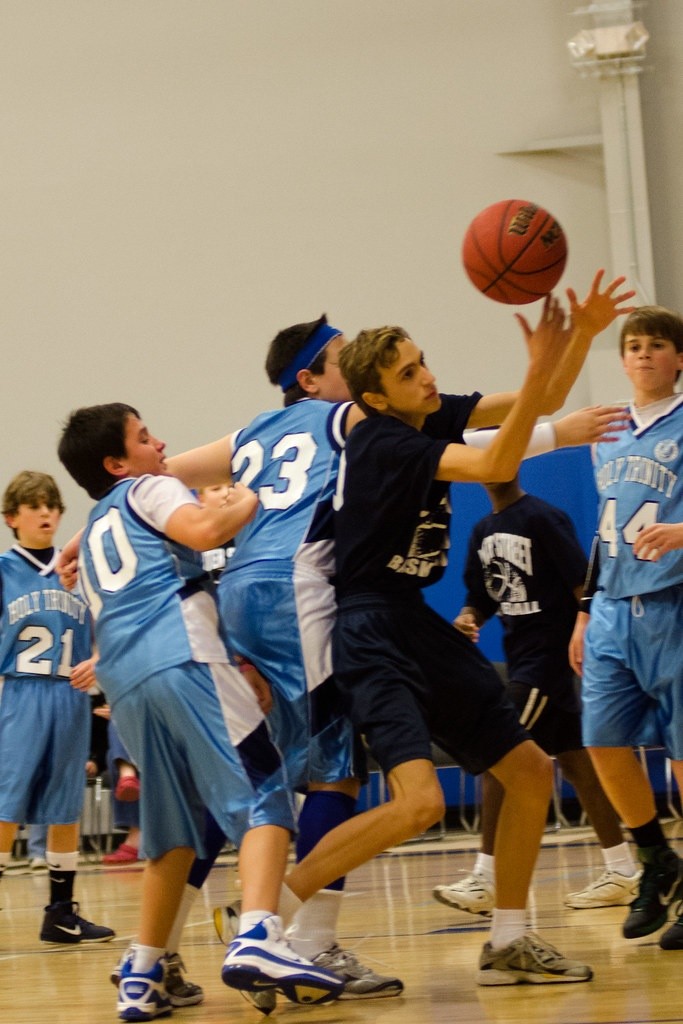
[464,200,568,305]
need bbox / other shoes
[102,845,144,866]
[28,857,47,870]
[113,776,139,802]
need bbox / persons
[0,267,683,1021]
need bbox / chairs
[77,746,683,866]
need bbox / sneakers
[117,959,173,1022]
[477,931,592,986]
[563,869,643,909]
[311,946,404,999]
[39,900,115,944]
[213,898,277,1015]
[432,869,494,917]
[622,853,683,949]
[111,948,204,1006]
[221,915,345,1004]
